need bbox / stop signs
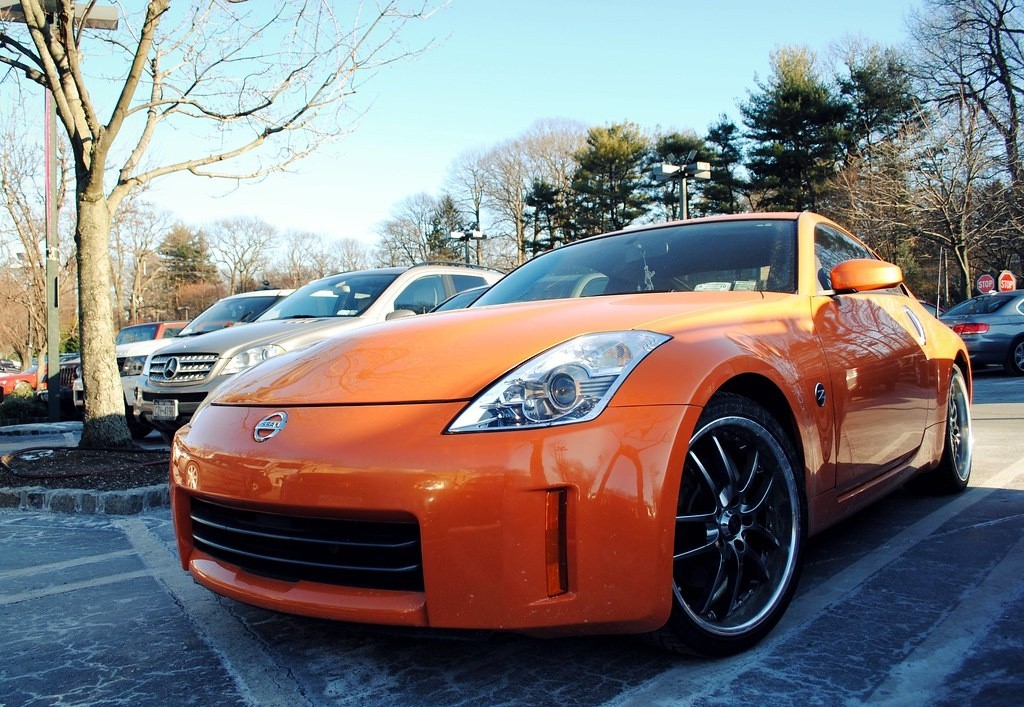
[976,274,994,294]
[998,270,1016,292]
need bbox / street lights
[177,306,190,322]
[653,148,711,220]
[446,222,487,268]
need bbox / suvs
[0,260,507,448]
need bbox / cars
[918,290,1024,376]
[171,213,974,654]
